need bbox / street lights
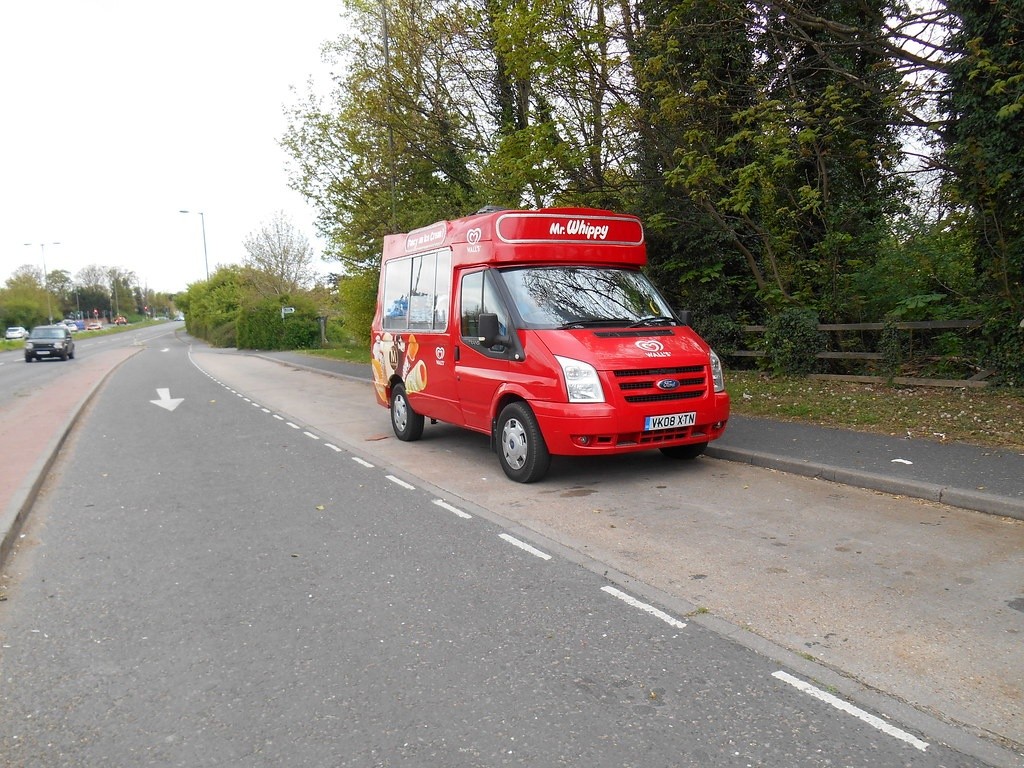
[180,210,209,285]
[24,243,61,325]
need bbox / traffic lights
[94,308,98,316]
[144,305,147,311]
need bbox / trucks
[370,206,730,484]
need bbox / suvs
[116,317,127,325]
[5,327,29,340]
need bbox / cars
[173,317,184,321]
[25,324,75,363]
[87,322,104,330]
[153,317,169,321]
[68,324,78,332]
[66,320,85,330]
[62,319,74,326]
[55,323,68,328]
